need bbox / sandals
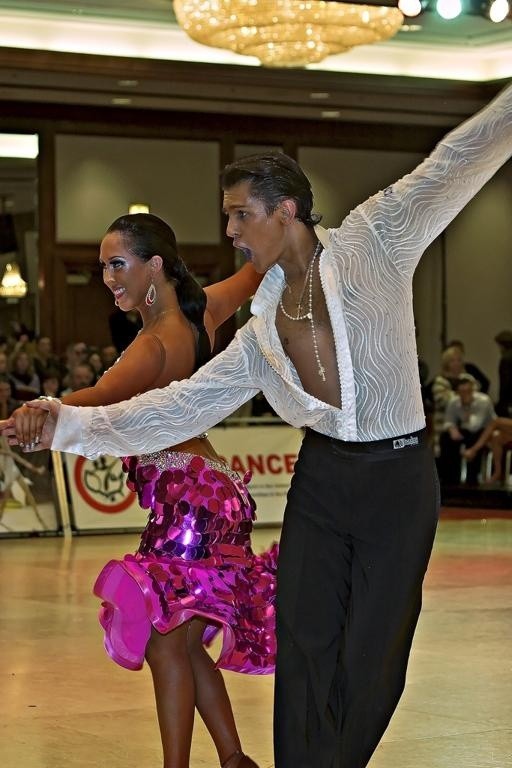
[221,751,258,768]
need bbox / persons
[109,308,143,354]
[2,81,512,768]
[14,211,266,767]
[418,330,511,485]
[1,322,119,501]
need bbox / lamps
[395,1,431,19]
[175,3,405,68]
[466,1,510,22]
[434,1,464,22]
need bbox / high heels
[486,477,503,488]
[459,446,478,462]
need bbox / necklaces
[284,268,307,319]
[138,306,183,335]
[280,240,326,382]
